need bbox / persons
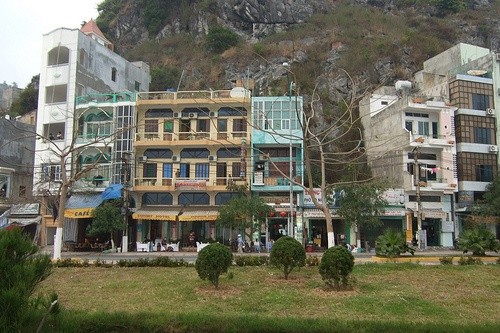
[175,169,181,177]
[254,239,261,253]
[187,230,195,252]
[228,174,234,186]
[143,237,149,243]
[162,237,167,250]
[236,235,245,253]
[344,244,352,252]
[267,238,275,253]
[50,132,63,140]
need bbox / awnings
[132,210,220,222]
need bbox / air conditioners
[189,112,198,118]
[208,155,217,161]
[259,154,268,160]
[486,108,494,115]
[209,111,218,118]
[488,145,498,152]
[172,155,180,162]
[139,156,147,161]
[173,112,182,118]
